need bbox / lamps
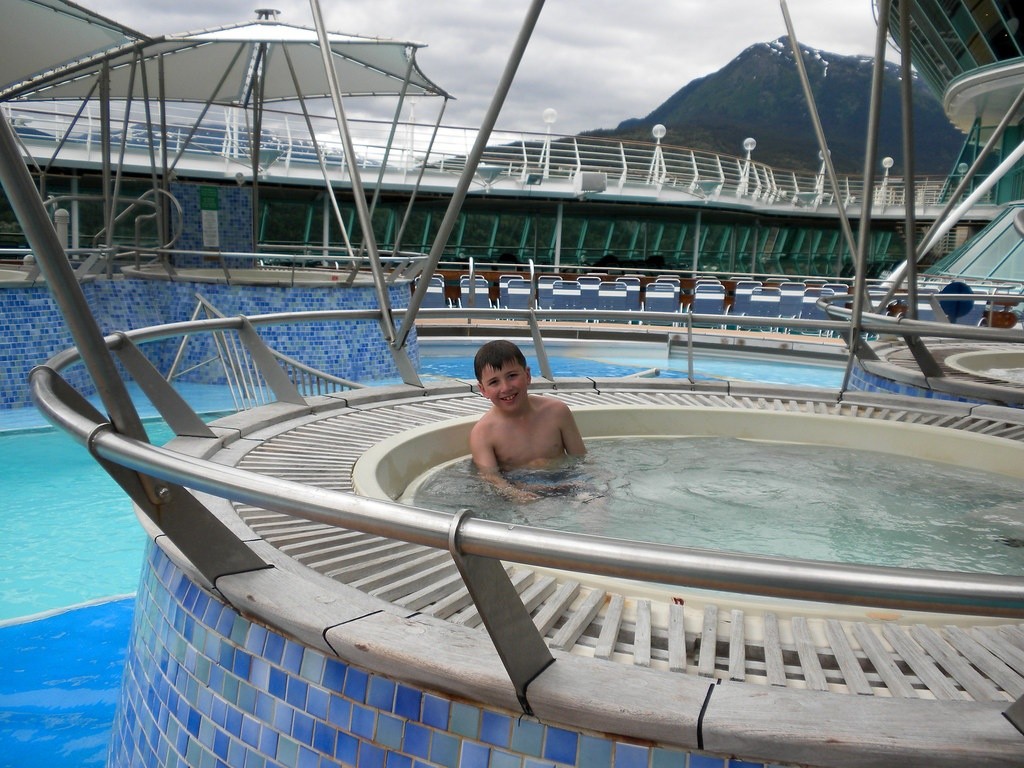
[526,173,545,187]
[542,108,558,123]
[818,148,830,159]
[882,157,894,169]
[743,138,756,151]
[654,125,667,139]
[571,171,609,193]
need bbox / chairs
[414,273,895,337]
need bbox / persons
[470,340,588,505]
